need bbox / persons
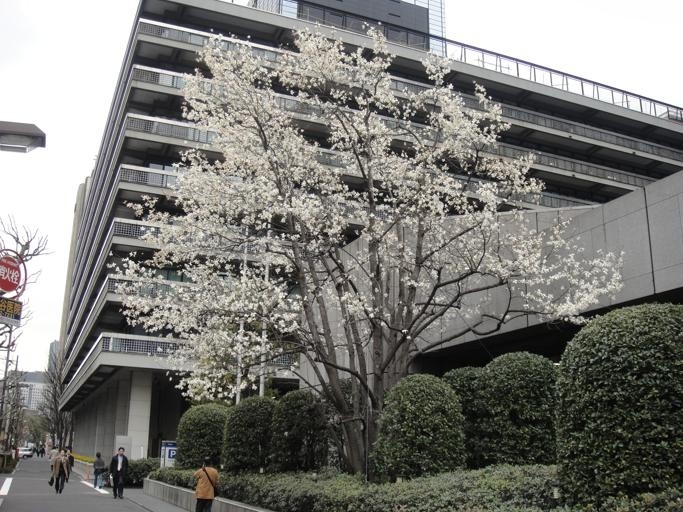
[51,449,69,494]
[49,446,58,472]
[92,452,105,489]
[192,456,220,511]
[64,449,74,483]
[32,440,46,458]
[110,447,128,500]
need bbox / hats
[16,446,32,459]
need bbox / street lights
[213,485,220,497]
[47,476,54,486]
[109,473,114,487]
